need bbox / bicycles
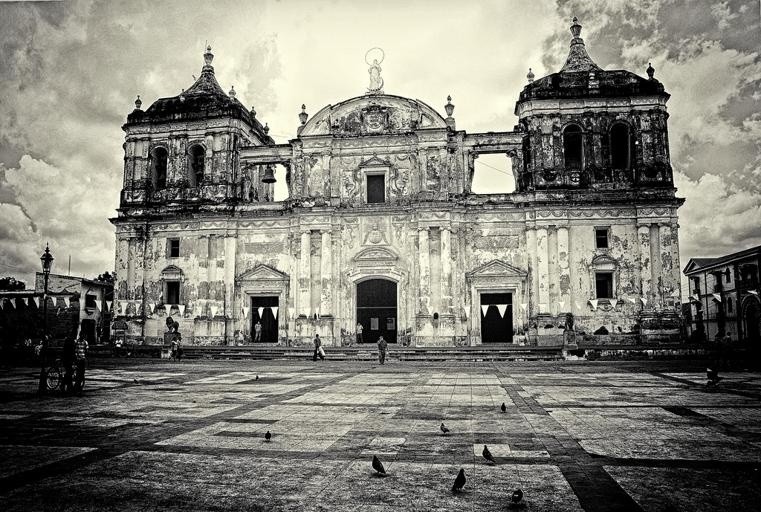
[45,357,85,391]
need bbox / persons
[252,320,263,343]
[177,336,185,363]
[355,322,365,344]
[57,331,75,395]
[715,329,739,367]
[313,333,326,361]
[72,330,91,393]
[168,336,179,362]
[23,332,54,359]
[376,335,391,365]
[110,334,125,358]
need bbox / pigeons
[440,423,449,433]
[451,468,466,491]
[501,403,506,412]
[265,431,271,442]
[511,489,523,502]
[373,455,386,474]
[483,445,496,463]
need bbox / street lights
[40,241,55,320]
